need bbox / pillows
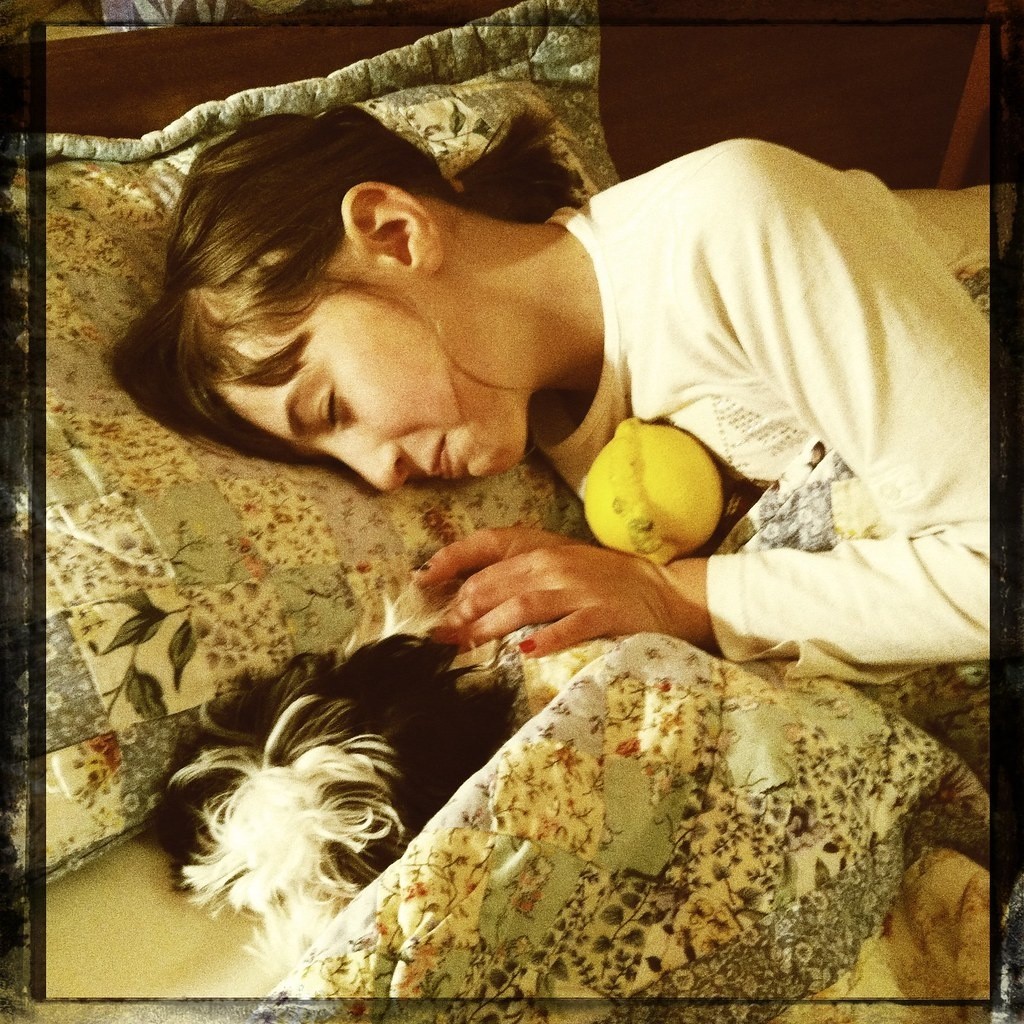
[1,1,623,888]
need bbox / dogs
[136,615,529,981]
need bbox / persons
[115,101,990,686]
